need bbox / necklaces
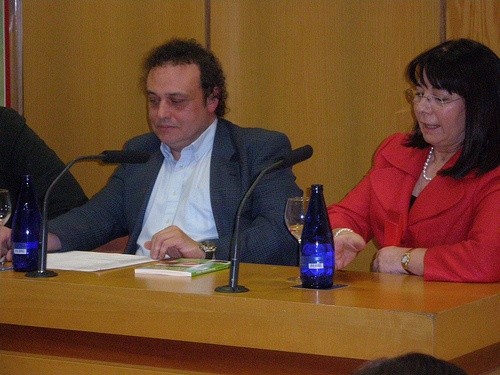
[423,146,434,180]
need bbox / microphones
[37,150,150,274]
[228,145,313,287]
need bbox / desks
[0,249,500,375]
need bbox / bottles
[11,174,41,271]
[299,183,335,289]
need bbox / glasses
[405,88,464,109]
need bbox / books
[134,257,232,277]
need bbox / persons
[326,38,500,281]
[0,107,90,231]
[0,37,300,265]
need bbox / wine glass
[0,189,12,271]
[285,197,310,282]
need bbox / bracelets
[333,228,354,239]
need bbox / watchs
[199,240,217,260]
[401,248,416,275]
